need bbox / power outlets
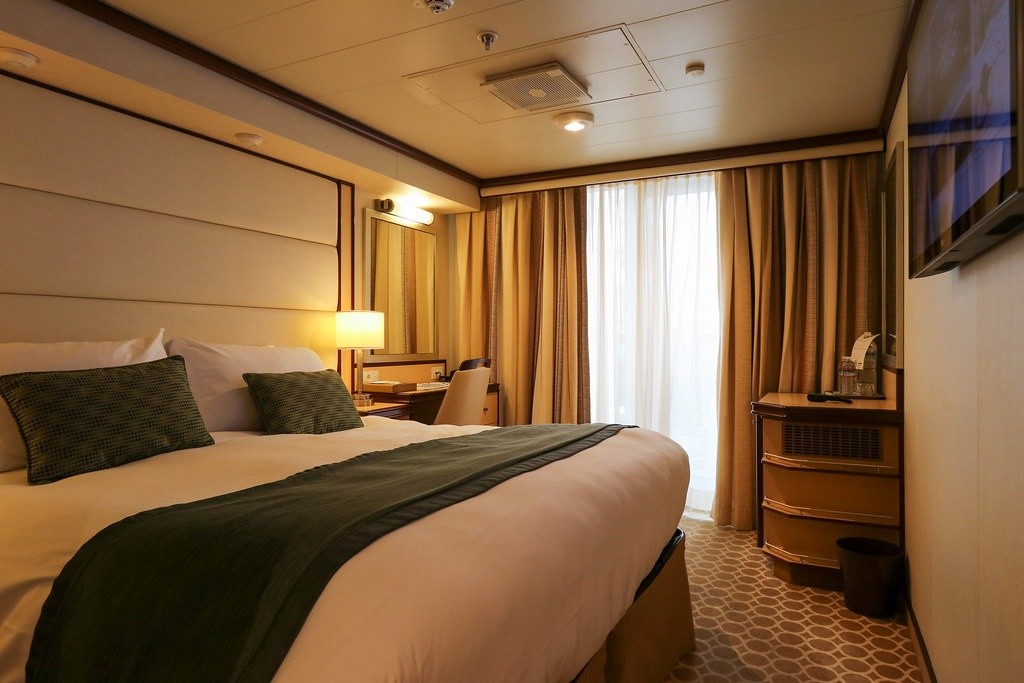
[431,367,444,379]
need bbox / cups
[857,382,875,395]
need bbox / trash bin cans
[836,536,905,617]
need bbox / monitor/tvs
[907,0,1024,281]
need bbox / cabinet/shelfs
[751,364,902,587]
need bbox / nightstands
[357,401,414,420]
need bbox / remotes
[807,394,853,404]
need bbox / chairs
[432,357,492,425]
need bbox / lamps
[376,197,436,225]
[554,111,594,132]
[335,310,385,408]
[480,63,592,111]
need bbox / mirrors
[363,208,438,357]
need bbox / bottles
[838,357,847,396]
[859,332,877,395]
[841,357,855,396]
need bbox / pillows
[2,328,167,473]
[164,339,328,431]
[1,353,213,485]
[243,370,366,435]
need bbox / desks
[355,359,501,427]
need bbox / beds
[1,338,698,682]
